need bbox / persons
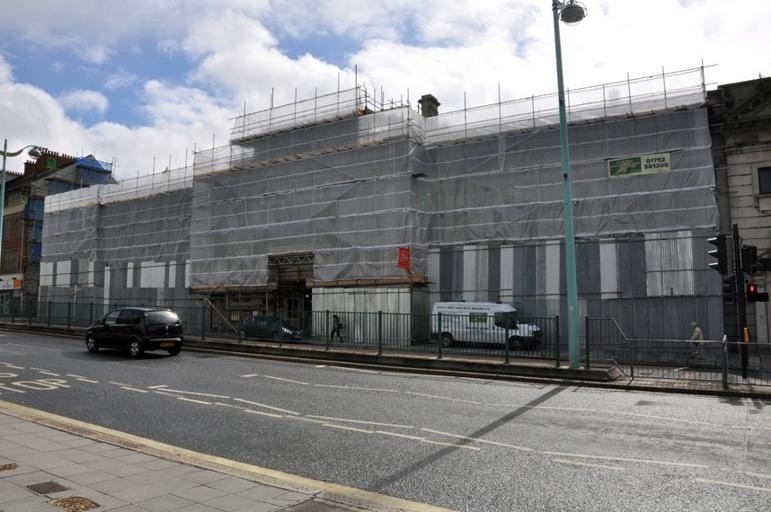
[688,321,707,360]
[329,313,346,344]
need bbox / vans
[84,307,184,357]
[431,302,542,350]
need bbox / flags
[398,247,412,272]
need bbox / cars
[239,316,304,341]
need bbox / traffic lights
[746,285,758,302]
[706,235,727,277]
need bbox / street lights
[552,0,587,368]
[0,137,42,250]
